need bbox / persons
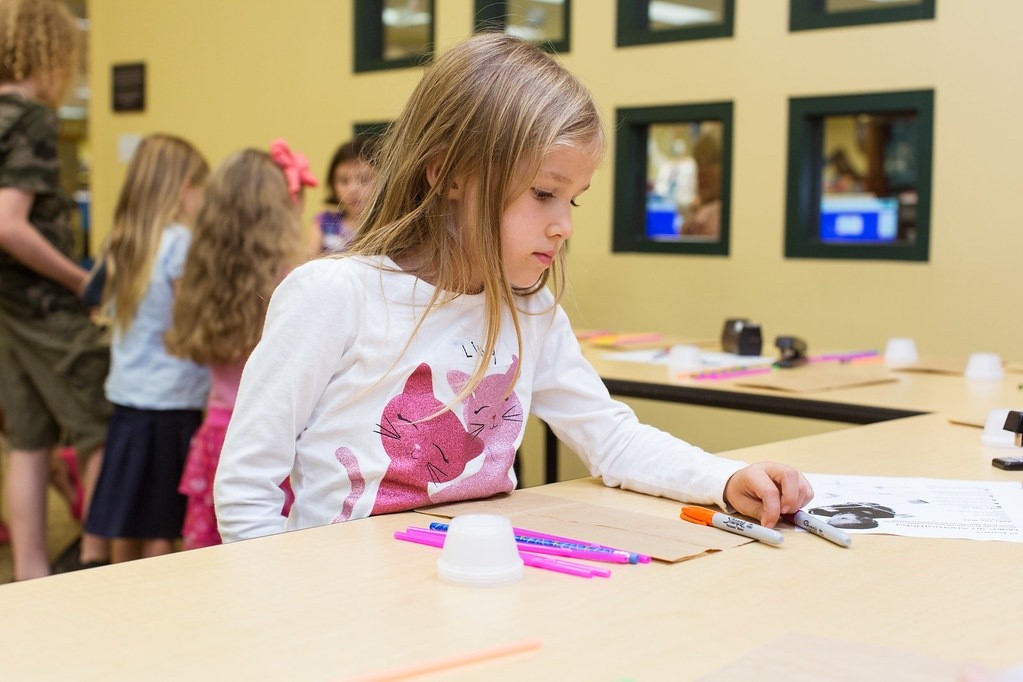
[213,33,813,545]
[809,501,895,529]
[306,140,387,258]
[0,0,107,582]
[165,148,316,550]
[82,132,211,560]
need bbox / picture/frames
[779,87,941,260]
[608,100,735,259]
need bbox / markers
[680,506,784,544]
[593,334,660,346]
[780,510,851,547]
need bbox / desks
[1,334,1021,679]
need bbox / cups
[434,515,524,579]
[964,352,1004,379]
[886,338,916,364]
[981,409,1015,447]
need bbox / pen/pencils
[429,522,639,564]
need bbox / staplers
[772,337,807,369]
[992,411,1023,470]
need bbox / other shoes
[49,448,89,523]
